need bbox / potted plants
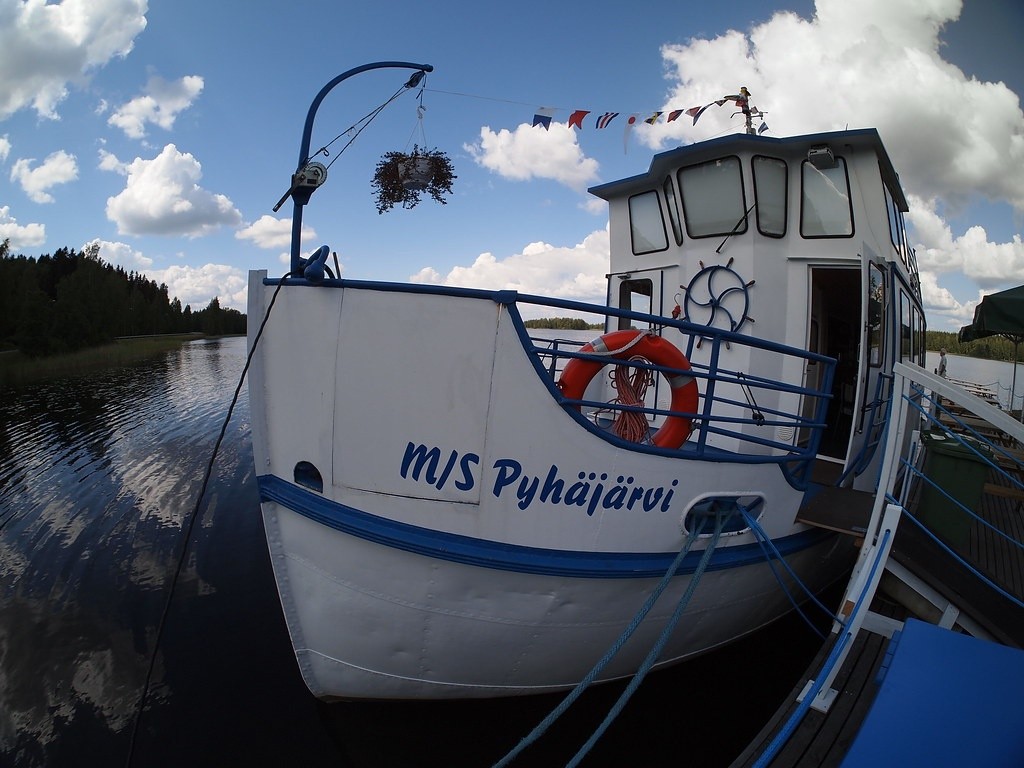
[370,144,458,215]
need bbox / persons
[937,348,947,378]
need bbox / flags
[668,110,683,122]
[693,103,714,126]
[568,110,590,129]
[532,107,555,131]
[717,100,727,106]
[751,106,758,114]
[758,122,769,134]
[596,112,619,129]
[645,112,663,125]
[685,107,700,117]
[625,113,642,126]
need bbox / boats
[246,60,927,731]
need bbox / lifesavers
[556,328,700,451]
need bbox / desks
[932,380,1024,513]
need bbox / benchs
[939,411,982,419]
[981,481,1024,503]
[937,425,1014,443]
